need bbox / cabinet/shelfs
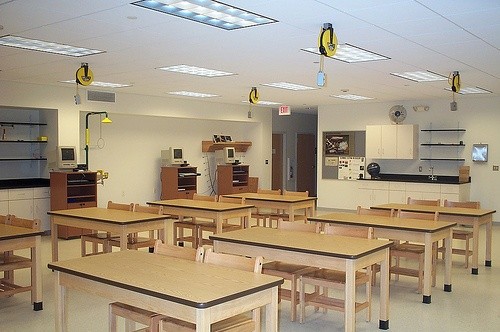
[0,122,48,161]
[420,129,466,161]
[217,165,249,200]
[161,166,197,219]
[49,171,98,240]
[366,124,417,160]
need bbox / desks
[222,193,318,222]
[46,208,172,272]
[145,199,256,254]
[47,249,284,332]
[209,227,395,332]
[369,203,497,275]
[0,223,45,312]
[307,213,458,304]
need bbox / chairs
[0,189,481,332]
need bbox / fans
[389,105,407,125]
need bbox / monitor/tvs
[160,147,184,167]
[215,147,235,164]
[47,145,78,172]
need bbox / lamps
[85,111,112,171]
